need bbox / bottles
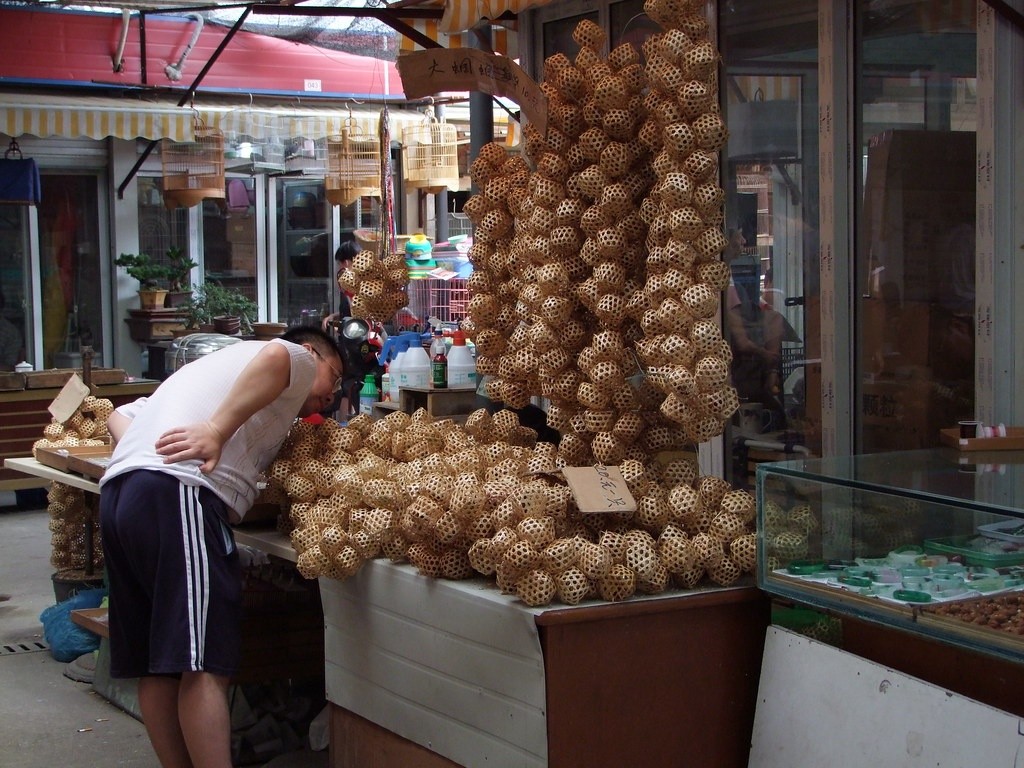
[359,375,379,416]
[428,329,452,387]
[382,368,391,403]
[432,346,448,388]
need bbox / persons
[322,242,364,332]
[723,222,803,427]
[0,291,23,370]
[97,323,344,768]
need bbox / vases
[253,321,288,339]
[51,567,105,601]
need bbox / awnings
[401,0,550,60]
[0,93,432,143]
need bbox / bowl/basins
[448,234,468,243]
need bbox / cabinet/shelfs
[345,385,504,424]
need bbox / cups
[15,361,34,373]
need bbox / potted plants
[163,247,197,307]
[205,284,258,335]
[183,296,216,333]
[110,253,169,309]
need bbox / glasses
[312,345,342,394]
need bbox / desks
[3,456,301,563]
